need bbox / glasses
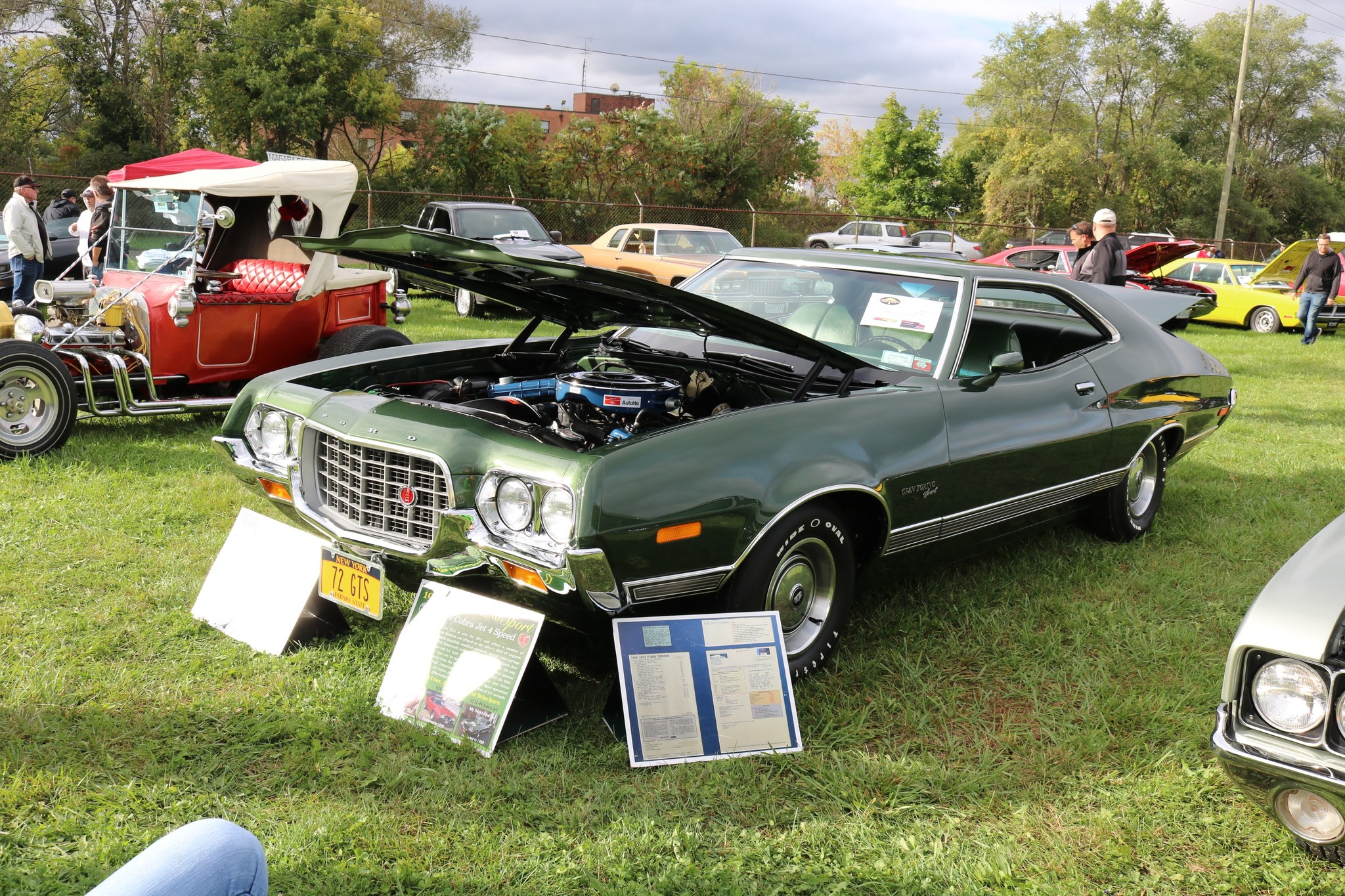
[1318,243,1330,247]
[1073,224,1087,238]
[20,186,36,191]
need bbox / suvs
[1,160,412,464]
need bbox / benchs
[625,242,683,255]
[929,310,1104,369]
[213,258,310,293]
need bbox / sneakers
[1310,327,1322,345]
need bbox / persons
[1076,208,1127,287]
[86,818,268,896]
[2,176,55,311]
[1271,246,1284,260]
[1066,220,1097,281]
[1196,245,1225,259]
[68,185,100,281]
[41,189,81,226]
[1290,233,1341,345]
[88,175,131,280]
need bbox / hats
[13,175,42,188]
[79,190,95,198]
[61,188,80,198]
[1093,208,1116,225]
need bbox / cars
[423,696,456,724]
[1210,511,1343,869]
[372,202,588,319]
[0,211,83,300]
[211,224,1235,681]
[558,228,825,318]
[460,716,492,738]
[805,222,1345,335]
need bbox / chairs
[782,303,856,346]
[957,324,1021,377]
